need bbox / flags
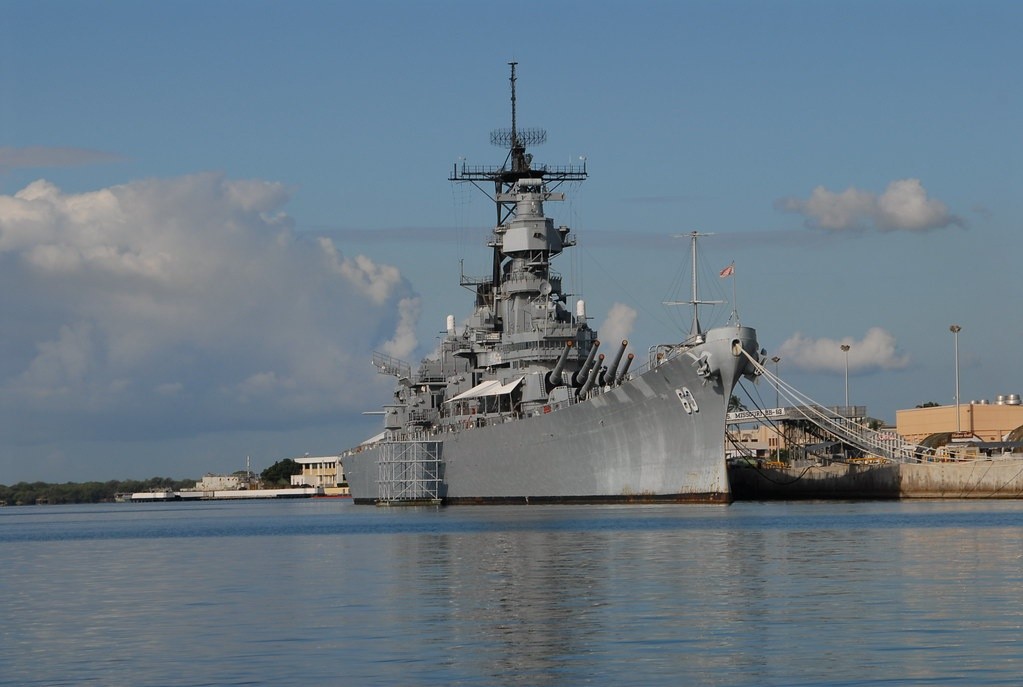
[720,262,735,278]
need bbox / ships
[339,61,758,508]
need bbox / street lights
[840,344,850,416]
[772,356,780,462]
[949,324,961,430]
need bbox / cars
[301,484,312,488]
